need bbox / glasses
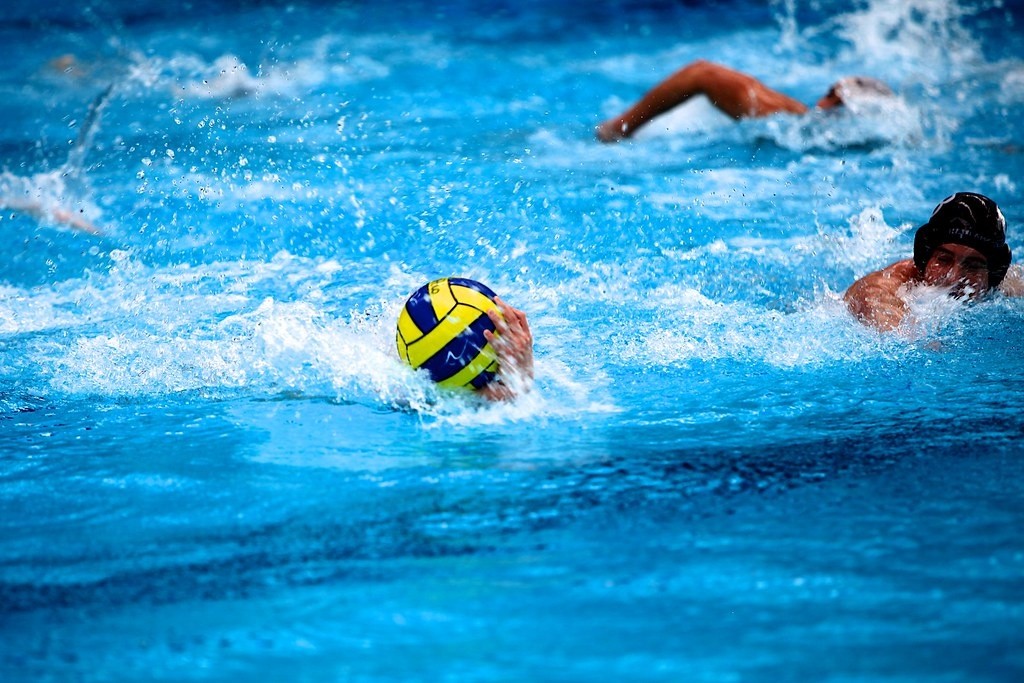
[826,86,842,105]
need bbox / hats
[834,77,894,102]
[913,191,1013,290]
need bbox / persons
[594,60,897,144]
[485,294,534,398]
[842,191,1012,336]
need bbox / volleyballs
[395,277,506,392]
